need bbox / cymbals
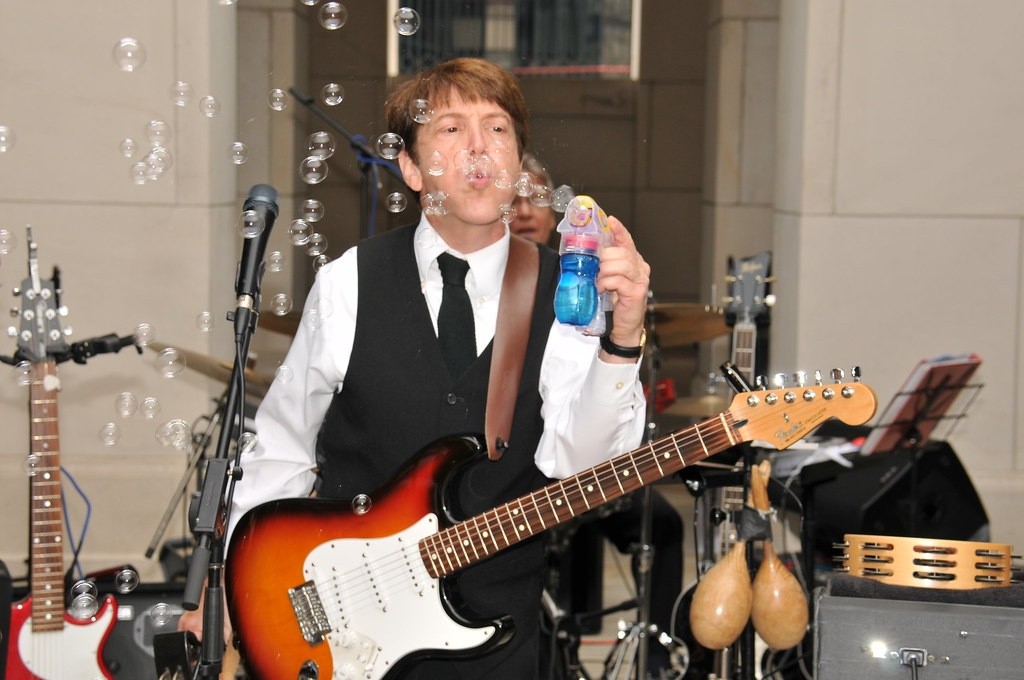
[147,340,273,400]
[640,302,733,350]
[253,308,302,339]
[831,540,1022,584]
[658,395,732,417]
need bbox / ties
[437,250,477,380]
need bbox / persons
[176,62,651,680]
[507,149,567,254]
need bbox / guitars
[4,225,118,680]
[726,251,778,405]
[224,362,878,680]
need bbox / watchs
[601,312,647,358]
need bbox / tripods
[609,327,689,680]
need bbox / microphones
[233,183,279,342]
